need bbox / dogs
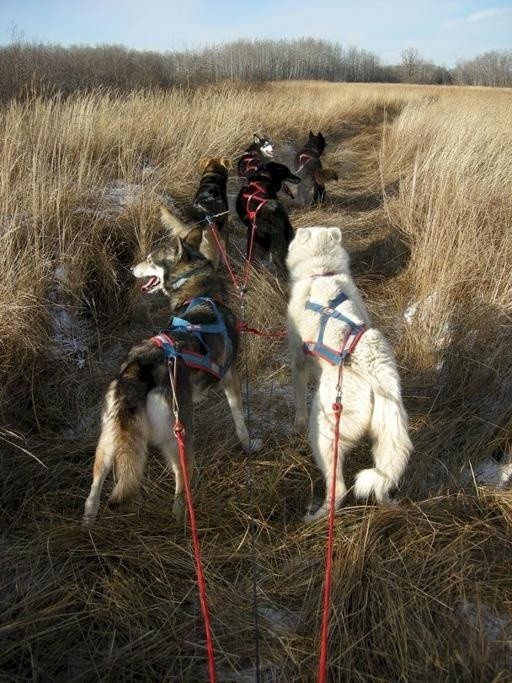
[159,155,231,272]
[236,133,277,179]
[293,128,339,208]
[280,224,415,525]
[235,161,303,281]
[77,223,265,537]
